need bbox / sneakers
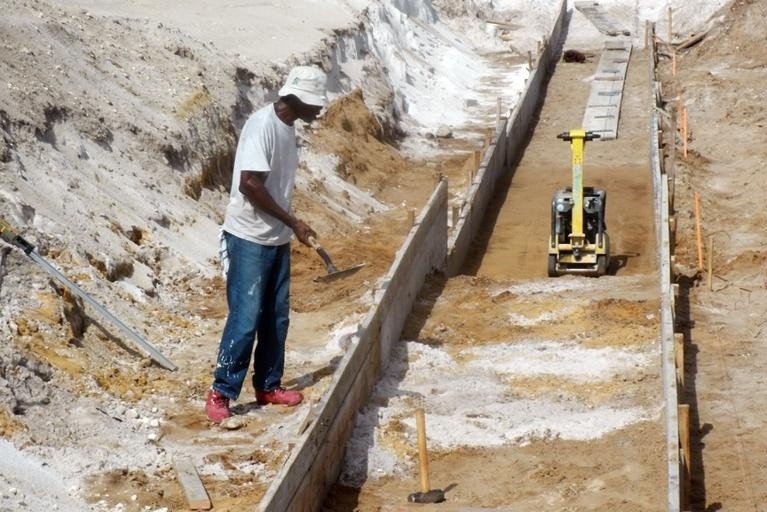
[204,389,230,423]
[256,389,303,405]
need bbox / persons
[205,65,331,424]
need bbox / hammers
[408,408,444,503]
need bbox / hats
[279,65,331,109]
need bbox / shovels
[308,234,367,283]
